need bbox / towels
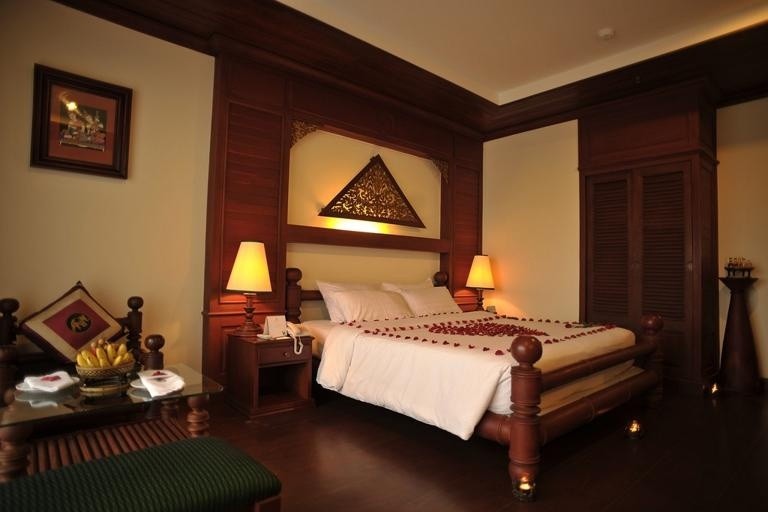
[22,370,75,395]
[138,368,188,402]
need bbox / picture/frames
[28,61,132,180]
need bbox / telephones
[287,321,311,337]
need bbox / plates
[15,376,80,392]
[127,388,151,399]
[130,379,147,390]
[15,388,75,403]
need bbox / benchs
[0,432,281,512]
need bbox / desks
[0,356,223,485]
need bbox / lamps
[463,254,494,312]
[317,151,426,231]
[226,240,272,335]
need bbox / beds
[285,265,662,501]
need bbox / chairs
[0,293,167,442]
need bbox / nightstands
[227,328,315,421]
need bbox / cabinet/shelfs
[577,103,718,400]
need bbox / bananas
[76,344,132,368]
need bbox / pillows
[315,280,380,324]
[328,289,412,324]
[20,278,124,367]
[397,285,463,317]
[381,277,433,290]
